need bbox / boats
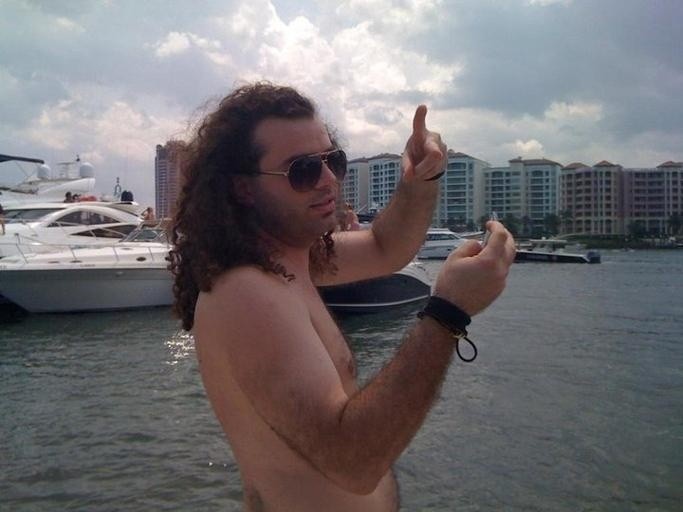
[514,238,602,263]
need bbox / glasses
[259,148,348,192]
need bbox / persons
[141,205,155,223]
[169,85,517,510]
[62,192,74,202]
[339,201,359,231]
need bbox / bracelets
[417,294,469,341]
[424,171,445,181]
